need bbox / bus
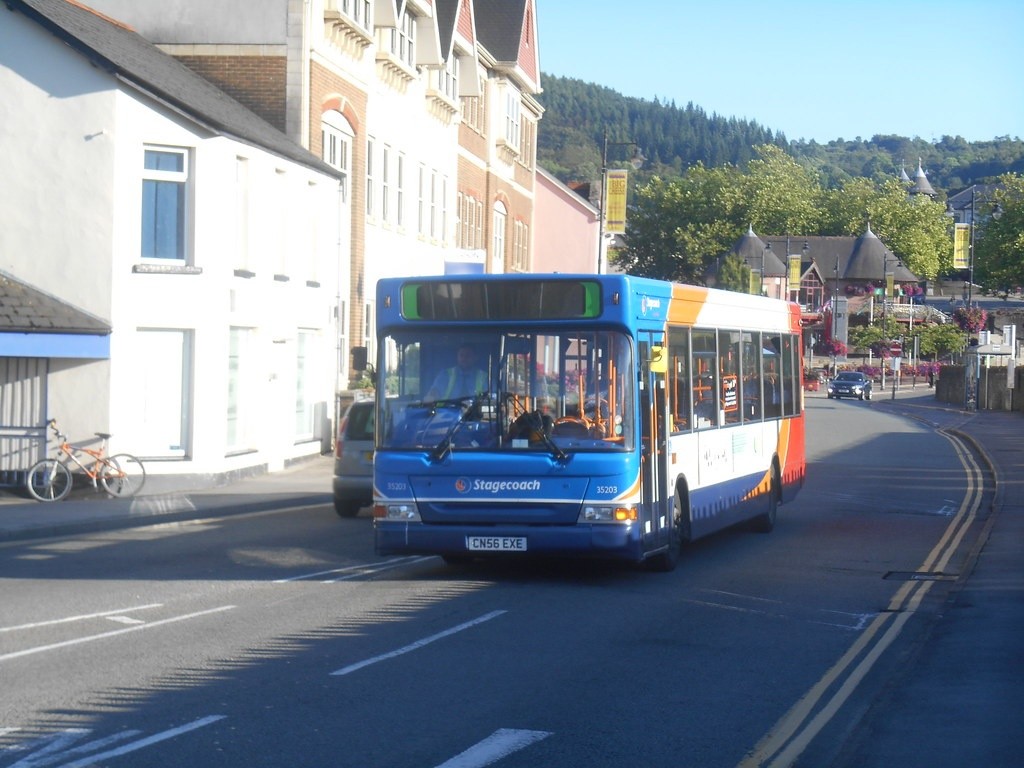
[351,273,808,573]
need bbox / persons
[929,368,934,388]
[423,343,498,404]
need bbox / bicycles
[25,418,146,503]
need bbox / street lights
[761,241,772,296]
[802,233,813,370]
[832,254,839,377]
[968,186,1005,308]
[881,251,903,390]
[597,128,646,274]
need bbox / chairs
[552,373,775,440]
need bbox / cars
[332,393,399,519]
[827,371,874,401]
[802,367,836,391]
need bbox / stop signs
[890,343,903,356]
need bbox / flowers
[901,284,923,296]
[951,306,988,334]
[823,338,849,357]
[864,283,874,296]
